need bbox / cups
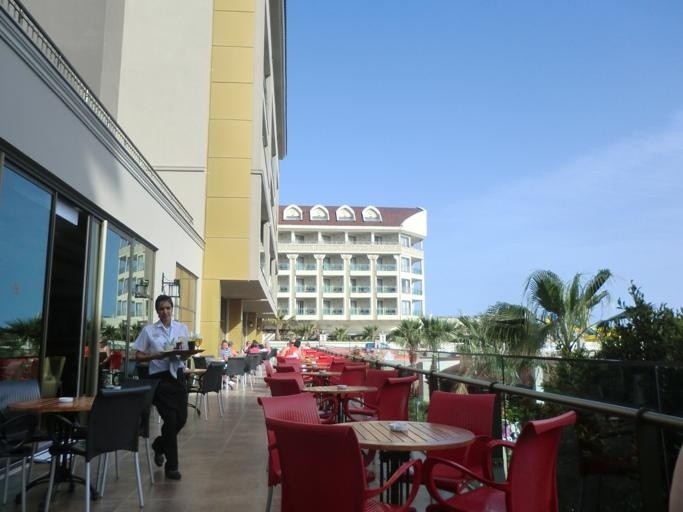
[176,342,182,350]
[188,338,195,350]
[178,336,186,349]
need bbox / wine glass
[194,337,202,350]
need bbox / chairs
[263,348,418,421]
[425,411,577,511]
[265,417,423,511]
[389,390,497,494]
[120,350,268,487]
[0,379,160,512]
[258,393,375,511]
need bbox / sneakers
[164,463,180,480]
[151,443,163,467]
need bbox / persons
[228,341,237,356]
[98,337,110,368]
[281,339,296,357]
[135,294,189,479]
[220,340,231,383]
[286,341,303,359]
[242,339,271,371]
